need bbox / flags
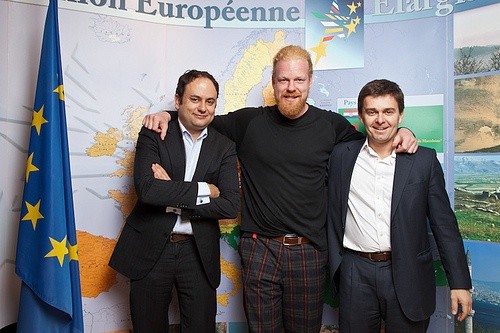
[15,0,84,333]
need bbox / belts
[271,234,310,246]
[342,246,392,263]
[169,232,193,244]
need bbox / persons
[142,46,419,333]
[328,79,474,333]
[109,69,241,333]
